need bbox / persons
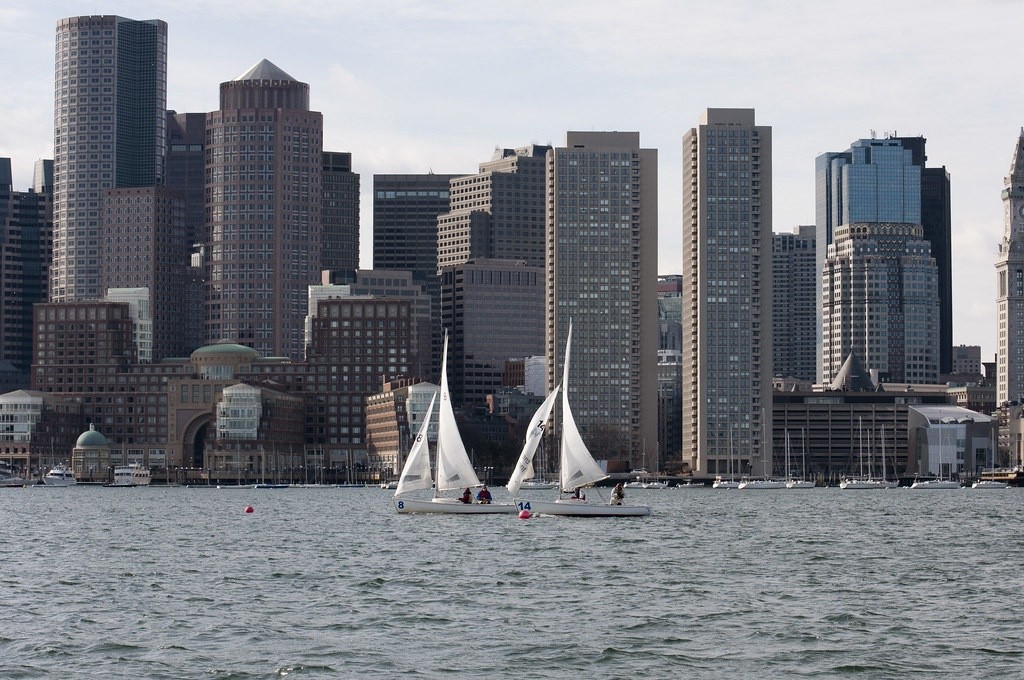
[459,488,471,504]
[476,485,492,505]
[571,487,588,500]
[610,483,625,507]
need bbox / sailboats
[838,416,899,489]
[712,427,739,488]
[506,316,651,517]
[623,439,648,488]
[641,440,670,489]
[147,440,384,487]
[971,426,1008,488]
[519,437,556,489]
[910,409,960,489]
[389,327,516,515]
[786,427,817,488]
[737,408,786,488]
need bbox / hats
[466,488,470,490]
[616,483,621,488]
[483,485,487,489]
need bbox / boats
[44,463,77,486]
[678,483,704,488]
[0,477,38,486]
[981,466,1024,487]
[114,458,152,485]
